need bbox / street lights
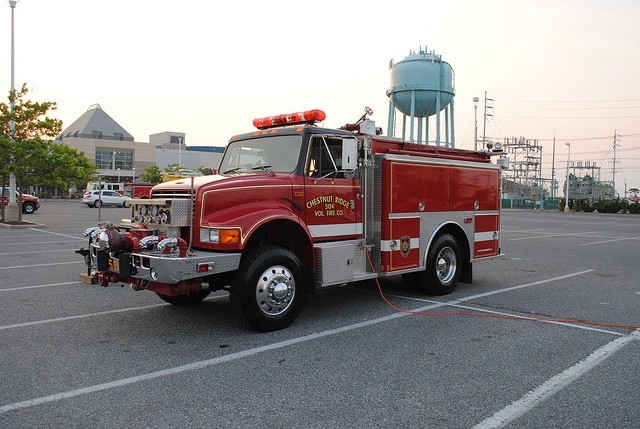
[178,136,182,166]
[118,168,121,182]
[4,0,22,222]
[473,97,479,151]
[133,168,136,183]
[564,142,570,212]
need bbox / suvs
[82,190,132,208]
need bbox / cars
[0,187,40,214]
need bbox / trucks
[86,181,124,191]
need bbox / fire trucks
[75,107,509,332]
[125,182,155,199]
